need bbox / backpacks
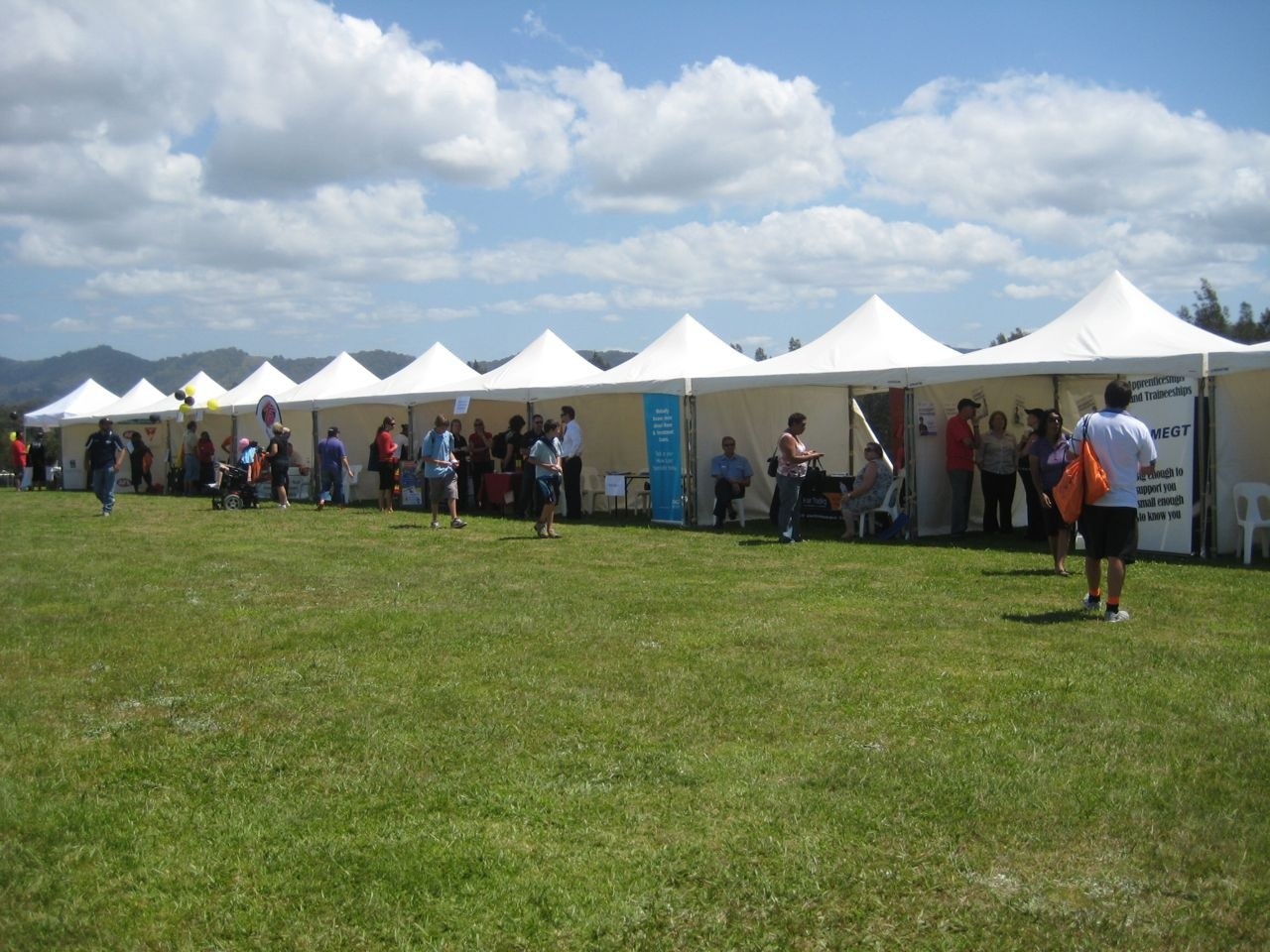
[492,430,513,459]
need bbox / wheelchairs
[211,462,256,510]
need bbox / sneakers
[1083,592,1130,624]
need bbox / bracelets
[848,492,852,499]
[436,460,439,466]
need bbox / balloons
[174,385,219,413]
[10,432,16,441]
[37,432,44,439]
[9,411,19,421]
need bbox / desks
[481,472,519,515]
[21,466,62,489]
[607,472,692,517]
[793,475,855,521]
[254,474,311,500]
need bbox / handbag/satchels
[368,441,380,472]
[143,451,152,474]
[174,443,184,469]
[768,445,778,478]
[1053,414,1111,524]
[804,457,827,492]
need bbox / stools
[633,491,651,515]
[713,497,746,527]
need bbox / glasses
[533,421,543,424]
[864,447,876,452]
[723,444,735,447]
[474,422,483,427]
[560,413,566,417]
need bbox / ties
[562,426,567,444]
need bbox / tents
[22,377,167,492]
[411,326,604,519]
[313,340,482,512]
[529,309,757,527]
[169,360,299,497]
[690,295,962,542]
[234,351,380,501]
[1208,339,1270,562]
[94,371,228,494]
[912,267,1250,559]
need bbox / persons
[316,427,353,511]
[376,416,567,539]
[710,413,893,541]
[181,421,293,509]
[29,432,46,491]
[12,429,29,491]
[85,418,154,519]
[947,376,1158,622]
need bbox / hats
[1024,408,1044,419]
[99,417,113,426]
[957,398,980,412]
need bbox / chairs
[1234,482,1270,563]
[859,477,906,538]
[343,465,362,504]
[584,468,612,515]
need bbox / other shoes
[714,507,735,532]
[103,511,110,517]
[278,502,291,508]
[317,503,324,510]
[431,518,467,527]
[208,484,220,489]
[533,523,562,539]
[1054,568,1071,577]
